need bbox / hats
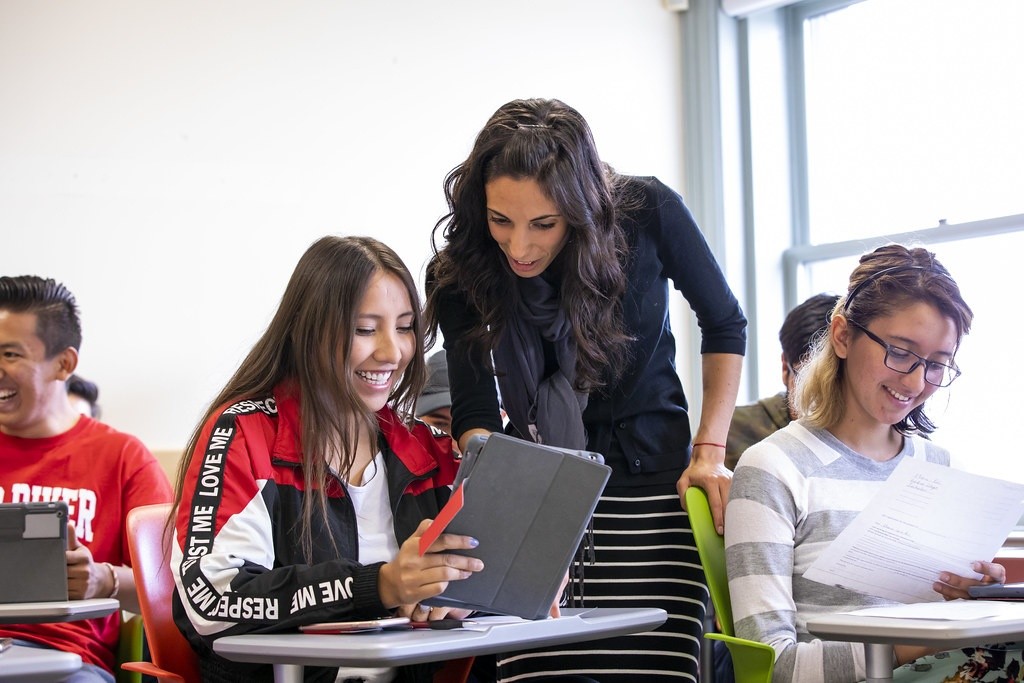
[414,350,452,418]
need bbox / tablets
[0,501,68,604]
[418,432,612,621]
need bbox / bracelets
[694,442,727,449]
[103,562,121,598]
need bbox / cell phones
[968,583,1024,598]
[299,617,410,630]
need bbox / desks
[0,643,83,683]
[212,607,670,683]
[0,597,120,624]
[806,597,1024,683]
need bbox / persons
[711,244,1024,683]
[414,98,747,683]
[161,234,485,683]
[0,275,178,683]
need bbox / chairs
[121,502,209,683]
[685,486,774,683]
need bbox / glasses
[845,317,961,387]
